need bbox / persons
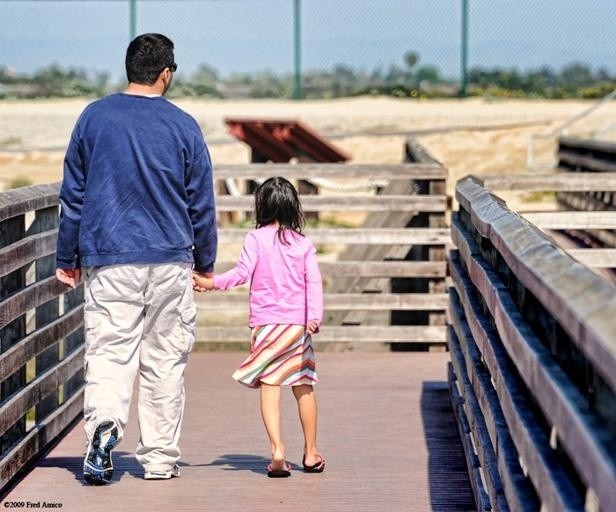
[192,176,325,476]
[53,32,218,488]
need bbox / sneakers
[143,456,181,480]
[82,418,120,487]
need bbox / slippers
[303,452,326,473]
[267,459,293,477]
